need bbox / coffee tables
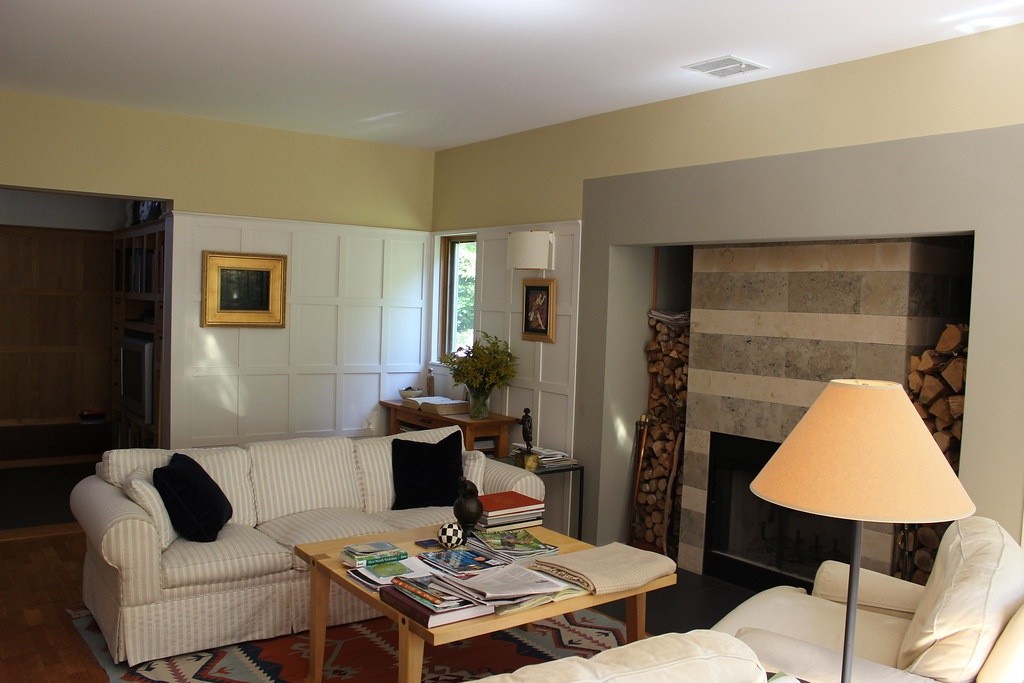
[295,522,678,683]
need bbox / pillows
[101,445,256,528]
[896,516,1024,683]
[462,449,485,496]
[122,462,180,553]
[242,436,364,525]
[352,425,466,514]
[152,452,233,543]
[391,430,463,511]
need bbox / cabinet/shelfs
[111,220,161,449]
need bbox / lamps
[507,230,558,272]
[749,378,977,683]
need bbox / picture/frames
[520,278,557,344]
[201,249,288,329]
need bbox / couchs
[709,559,1024,683]
[464,629,799,682]
[68,426,545,668]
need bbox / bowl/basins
[399,389,424,400]
[79,408,105,421]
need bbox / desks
[379,400,519,459]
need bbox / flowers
[438,331,521,393]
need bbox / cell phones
[415,539,440,549]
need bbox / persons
[516,407,533,449]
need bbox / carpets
[64,607,653,683]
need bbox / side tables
[492,457,585,541]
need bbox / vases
[464,380,498,419]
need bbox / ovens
[114,335,153,425]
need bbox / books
[402,396,469,416]
[379,586,494,629]
[342,491,559,615]
[509,442,578,468]
